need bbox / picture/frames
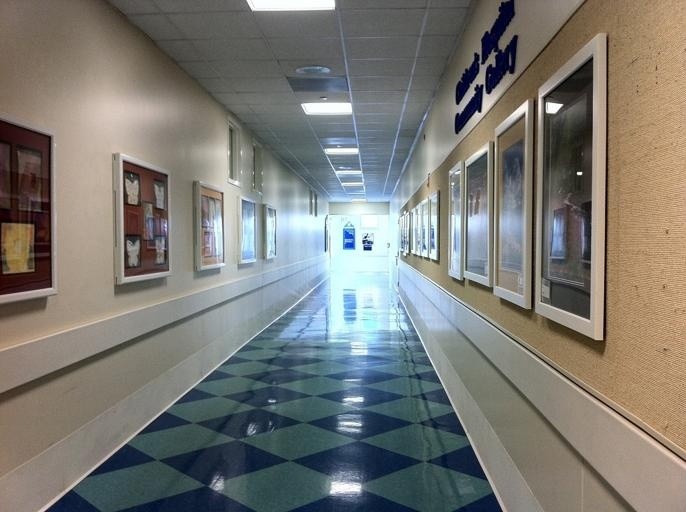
[110,150,174,287]
[396,187,440,264]
[493,98,533,314]
[461,140,494,292]
[192,180,227,273]
[447,156,463,284]
[532,28,610,345]
[237,194,260,266]
[0,113,59,308]
[261,203,278,262]
